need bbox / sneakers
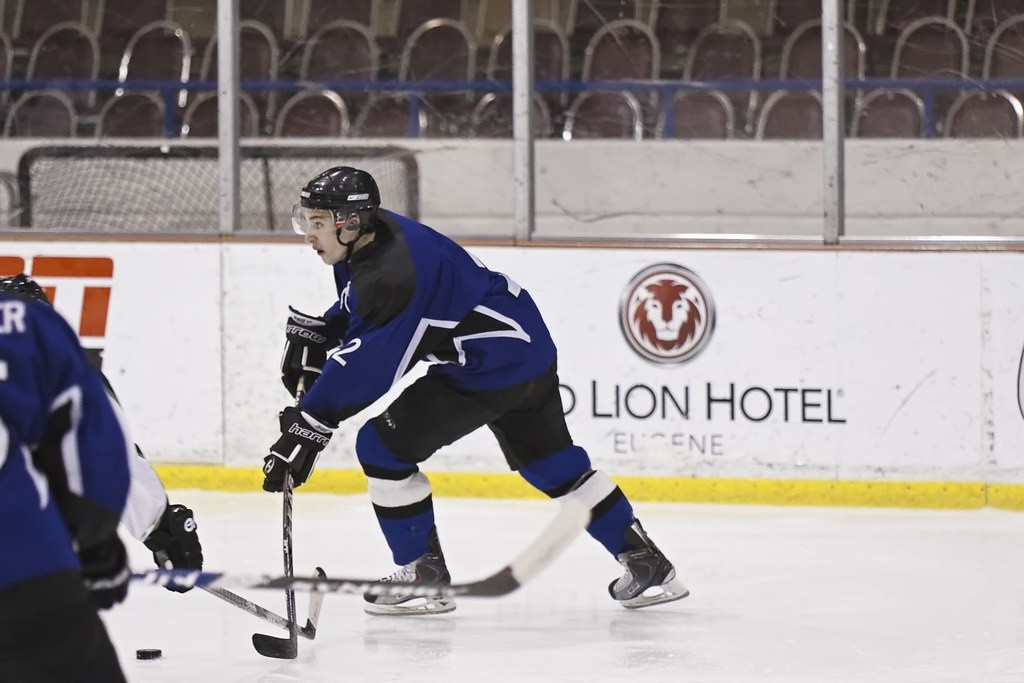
[363,537,457,617]
[608,519,689,609]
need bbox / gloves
[78,538,130,609]
[281,304,329,402]
[262,405,333,493]
[143,504,204,593]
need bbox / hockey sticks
[195,568,326,641]
[128,496,593,598]
[252,375,300,661]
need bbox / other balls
[136,648,162,660]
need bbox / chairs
[0,0,1024,141]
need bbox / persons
[262,167,689,616]
[0,273,203,683]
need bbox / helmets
[301,166,381,232]
